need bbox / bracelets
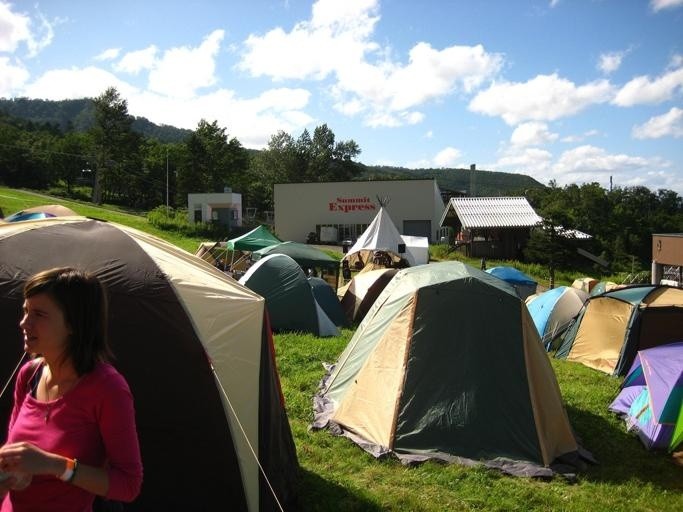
[56,457,80,485]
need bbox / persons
[0,267,148,512]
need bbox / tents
[196,206,420,338]
[0,204,80,224]
[0,211,308,512]
[308,259,595,480]
[486,262,682,458]
